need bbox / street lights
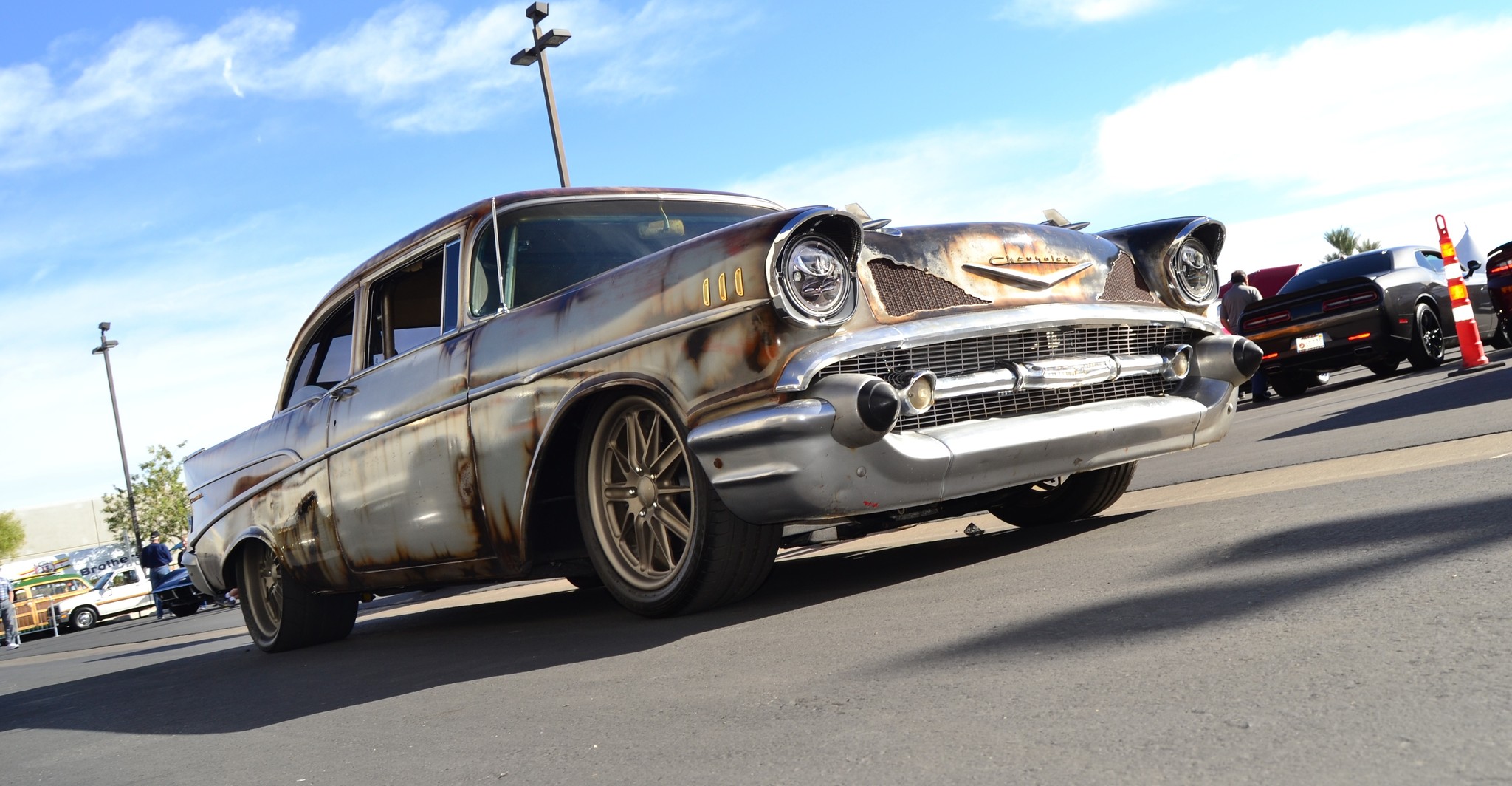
[92,322,146,578]
[510,3,573,191]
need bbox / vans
[11,575,93,604]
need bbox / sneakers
[6,643,19,650]
[213,594,235,607]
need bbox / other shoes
[170,612,175,616]
[157,616,164,619]
[1254,392,1270,403]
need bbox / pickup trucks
[176,190,1264,654]
[47,565,155,631]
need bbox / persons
[215,587,239,608]
[178,534,208,610]
[142,530,177,619]
[1220,270,1272,402]
[0,568,20,650]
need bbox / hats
[150,531,159,538]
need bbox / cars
[152,567,215,618]
[1219,240,1512,400]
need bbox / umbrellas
[169,541,184,552]
